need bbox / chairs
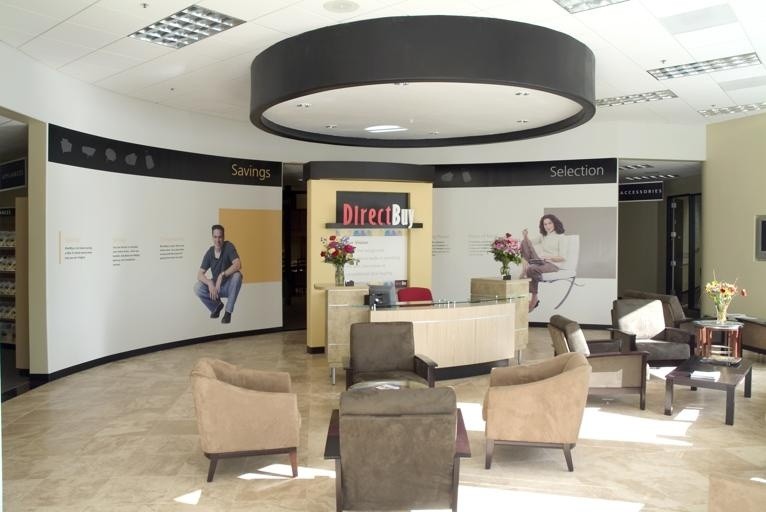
[397,287,434,307]
[541,233,580,309]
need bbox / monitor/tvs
[755,215,766,262]
[368,285,395,311]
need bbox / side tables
[693,319,745,358]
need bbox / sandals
[529,300,540,312]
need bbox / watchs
[221,271,226,281]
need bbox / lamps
[249,15,596,149]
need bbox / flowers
[320,236,361,267]
[487,232,524,266]
[705,269,749,304]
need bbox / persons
[193,224,244,324]
[518,214,569,313]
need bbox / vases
[335,265,344,287]
[713,303,729,325]
[500,266,512,279]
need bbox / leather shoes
[222,312,231,323]
[210,302,224,318]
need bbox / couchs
[548,314,651,411]
[343,321,439,392]
[336,387,460,512]
[481,352,593,473]
[189,358,303,483]
[619,299,698,392]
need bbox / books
[708,354,743,364]
[690,370,720,381]
